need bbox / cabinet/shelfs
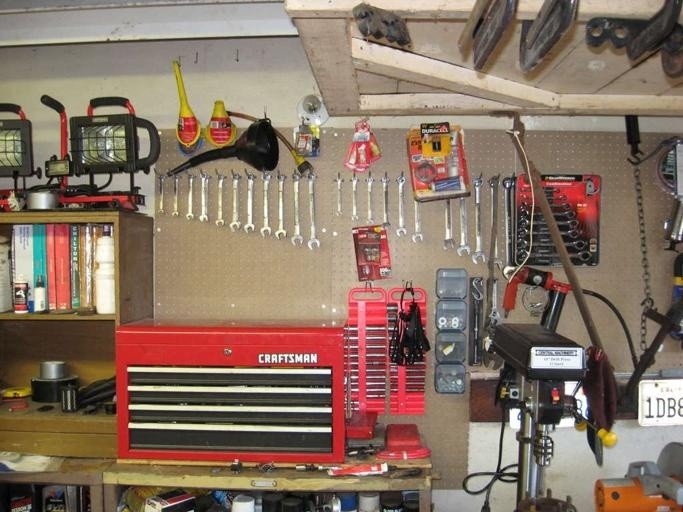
[101,457,436,510]
[0,211,154,456]
[0,458,115,512]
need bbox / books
[1,223,115,317]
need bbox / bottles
[94,231,115,315]
[34,275,46,313]
